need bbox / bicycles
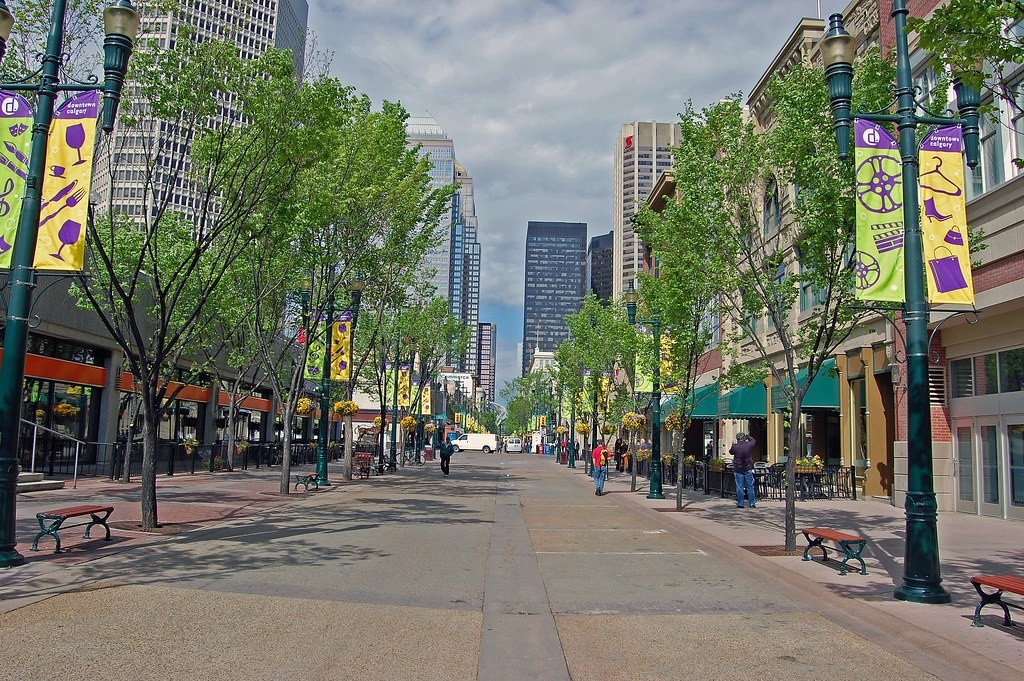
[395,448,427,467]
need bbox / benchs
[803,528,869,575]
[294,472,321,491]
[970,574,1024,627]
[358,462,397,478]
[30,505,114,554]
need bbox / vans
[506,438,523,454]
[451,433,500,454]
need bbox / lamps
[887,362,907,389]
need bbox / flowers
[796,454,825,467]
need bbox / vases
[796,465,823,473]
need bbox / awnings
[659,379,767,422]
[771,358,840,414]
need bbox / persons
[614,439,621,470]
[729,433,756,508]
[592,439,608,496]
[705,440,713,455]
[639,438,652,449]
[574,439,579,461]
[440,437,454,477]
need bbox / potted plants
[710,456,725,470]
[662,452,696,469]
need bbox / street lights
[625,245,666,500]
[299,263,364,487]
[379,336,417,472]
[824,1,984,604]
[0,1,141,569]
